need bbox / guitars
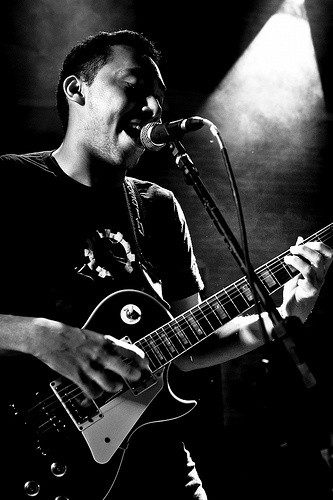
[0,223,333,500]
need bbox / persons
[1,27,333,500]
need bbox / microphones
[138,117,210,152]
